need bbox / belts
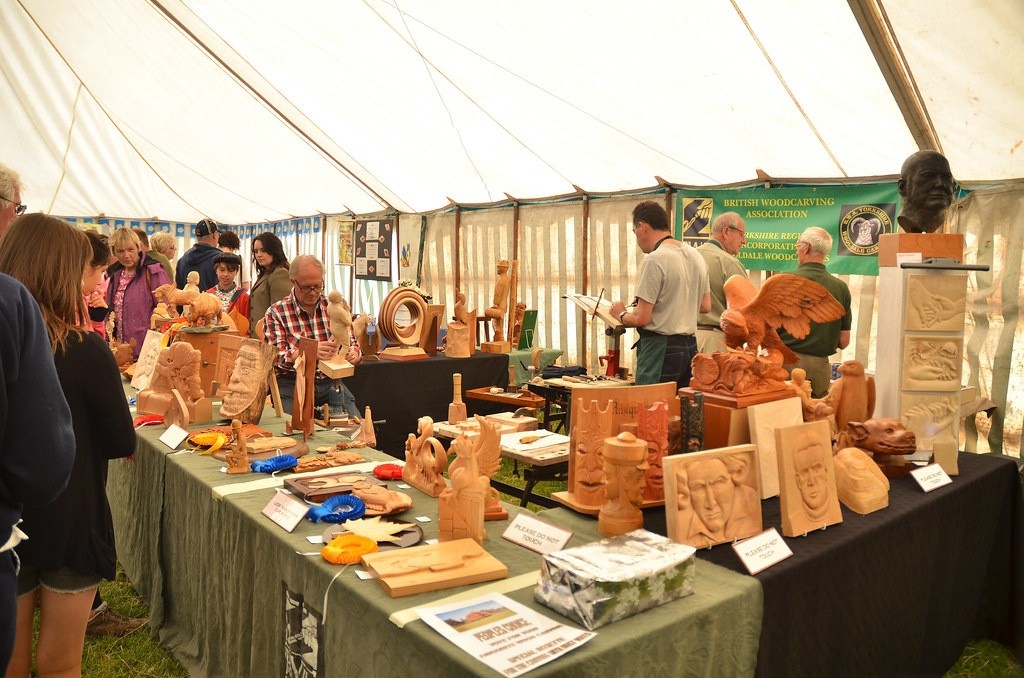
[697,325,723,332]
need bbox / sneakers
[86,601,148,636]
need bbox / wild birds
[442,413,502,506]
[720,274,845,361]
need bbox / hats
[212,252,242,265]
[195,219,222,237]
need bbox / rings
[352,355,355,359]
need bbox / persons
[599,368,843,551]
[610,200,711,396]
[897,149,954,233]
[492,260,511,341]
[183,271,201,314]
[777,227,852,399]
[0,167,364,678]
[220,340,278,425]
[695,212,748,355]
[326,290,352,361]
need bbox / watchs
[619,311,629,323]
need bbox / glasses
[728,226,744,237]
[293,279,325,293]
[794,241,811,250]
[0,197,27,216]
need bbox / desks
[640,450,1024,678]
[110,368,764,678]
[474,345,564,390]
[342,347,509,462]
[432,415,571,508]
